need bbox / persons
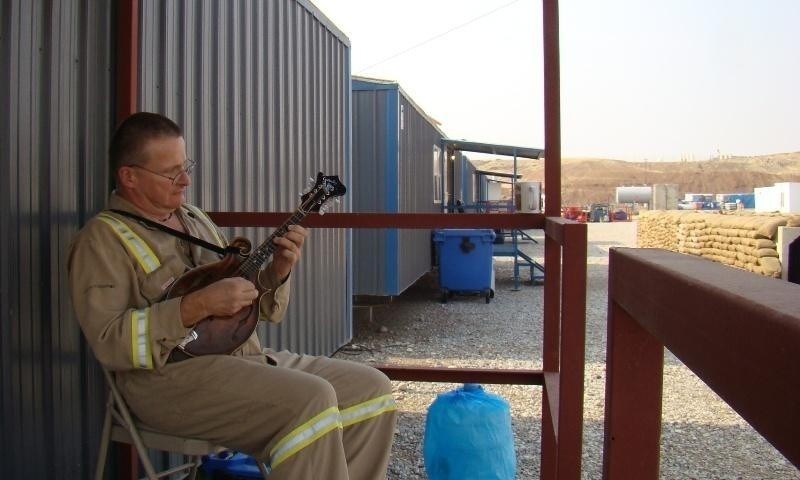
[69,113,398,480]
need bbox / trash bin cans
[434,227,496,304]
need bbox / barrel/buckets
[197,449,269,480]
[424,380,516,478]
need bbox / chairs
[95,363,217,479]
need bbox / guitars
[166,170,346,357]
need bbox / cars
[616,208,627,220]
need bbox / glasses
[128,159,198,185]
